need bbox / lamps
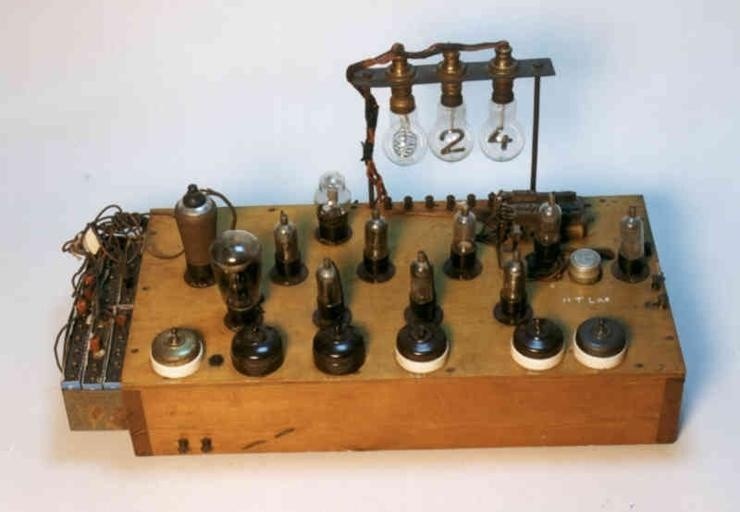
[381,41,525,166]
[314,171,354,244]
[208,228,264,330]
